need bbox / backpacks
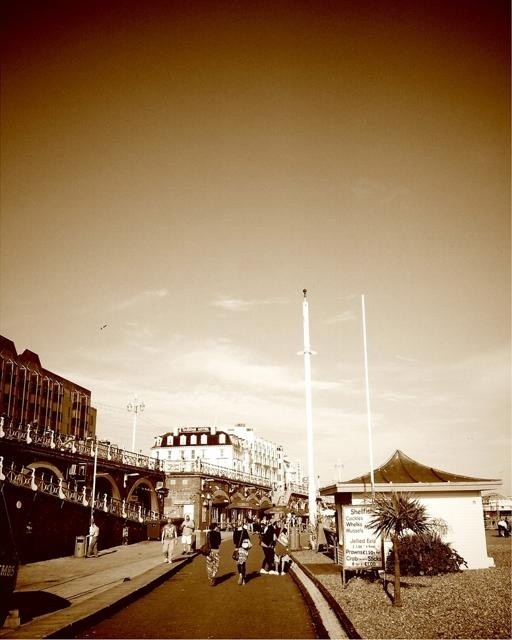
[232,550,239,561]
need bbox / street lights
[126,395,145,451]
[90,439,110,546]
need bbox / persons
[507,522,512,535]
[498,520,510,538]
[203,522,222,586]
[244,513,305,534]
[232,518,252,586]
[260,518,281,575]
[161,517,178,564]
[273,518,290,577]
[179,514,196,556]
[121,522,130,549]
[84,520,100,559]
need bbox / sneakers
[259,568,287,576]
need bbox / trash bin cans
[74,535,86,557]
[289,527,300,551]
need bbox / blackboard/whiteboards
[342,505,385,570]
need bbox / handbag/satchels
[200,544,211,556]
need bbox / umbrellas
[224,499,262,511]
[264,505,291,514]
